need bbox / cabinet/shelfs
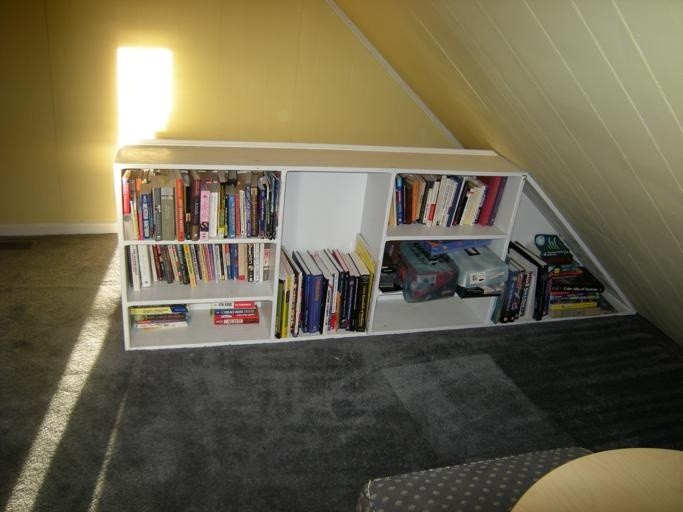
[112,140,637,352]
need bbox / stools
[357,446,589,512]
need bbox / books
[119,168,281,332]
[492,240,616,323]
[275,234,378,338]
[387,174,507,231]
[377,239,504,299]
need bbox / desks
[508,448,682,512]
